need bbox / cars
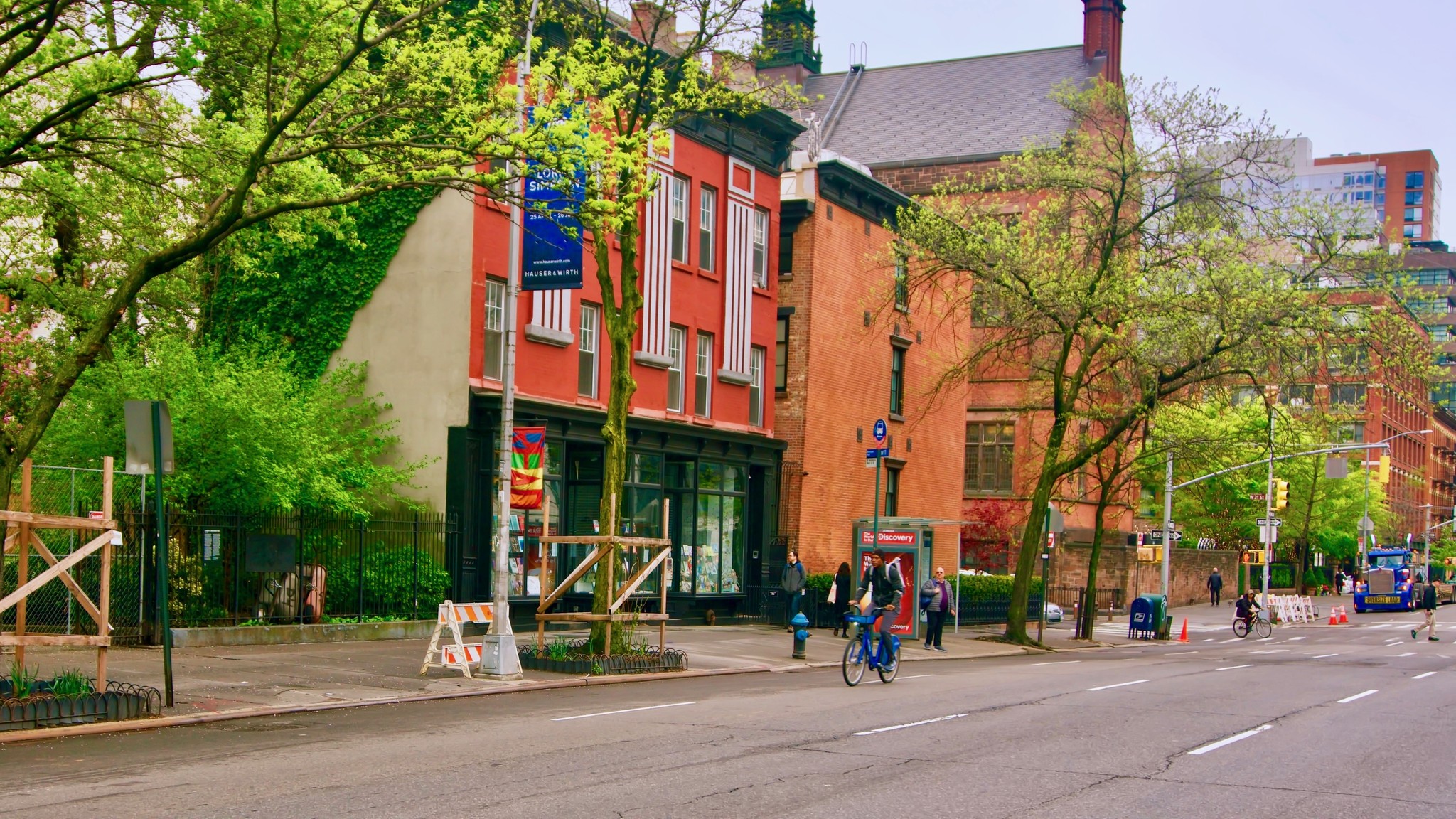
[1039,600,1064,625]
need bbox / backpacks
[869,557,905,588]
[1236,598,1244,607]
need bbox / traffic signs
[1268,510,1275,518]
[1167,520,1175,531]
[1255,518,1282,527]
[1151,529,1183,541]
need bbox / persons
[1410,578,1440,640]
[1207,568,1223,607]
[832,562,851,638]
[1255,566,1359,596]
[781,551,808,633]
[846,549,904,671]
[1236,589,1264,633]
[921,566,956,653]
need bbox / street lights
[1361,429,1434,569]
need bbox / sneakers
[1411,629,1417,639]
[1428,636,1439,641]
[787,625,794,633]
[884,656,897,671]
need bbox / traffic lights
[1276,481,1290,509]
[1242,551,1256,564]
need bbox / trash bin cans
[1153,616,1173,640]
[1129,597,1153,630]
[1139,592,1167,633]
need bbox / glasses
[937,572,942,574]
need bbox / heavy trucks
[1353,539,1456,614]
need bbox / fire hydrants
[790,611,813,659]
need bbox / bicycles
[841,603,902,687]
[1233,609,1272,638]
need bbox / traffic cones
[1338,604,1349,623]
[1176,617,1190,642]
[1328,605,1339,625]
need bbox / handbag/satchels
[826,573,837,605]
[920,578,936,615]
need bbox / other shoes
[934,645,947,652]
[842,634,849,638]
[924,642,931,650]
[833,628,838,636]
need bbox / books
[494,513,718,597]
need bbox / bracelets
[1261,608,1262,609]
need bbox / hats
[1431,577,1440,582]
[1247,590,1253,594]
[872,549,886,563]
